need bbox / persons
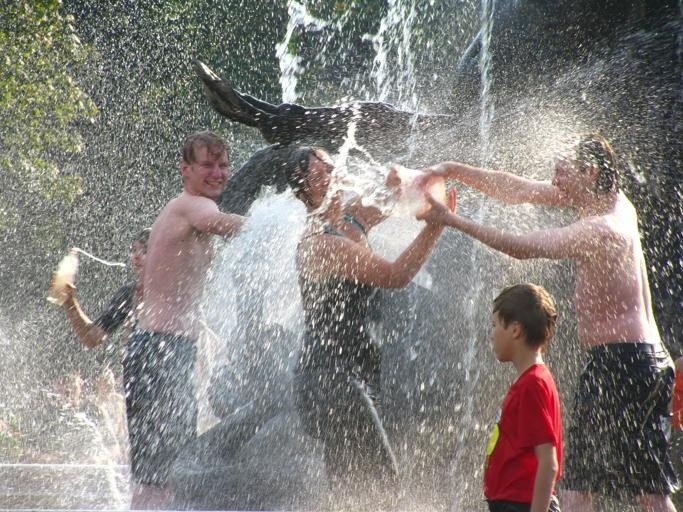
[409,135,680,512]
[483,280,565,510]
[119,130,251,511]
[53,228,152,349]
[286,144,459,512]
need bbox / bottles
[43,245,82,309]
